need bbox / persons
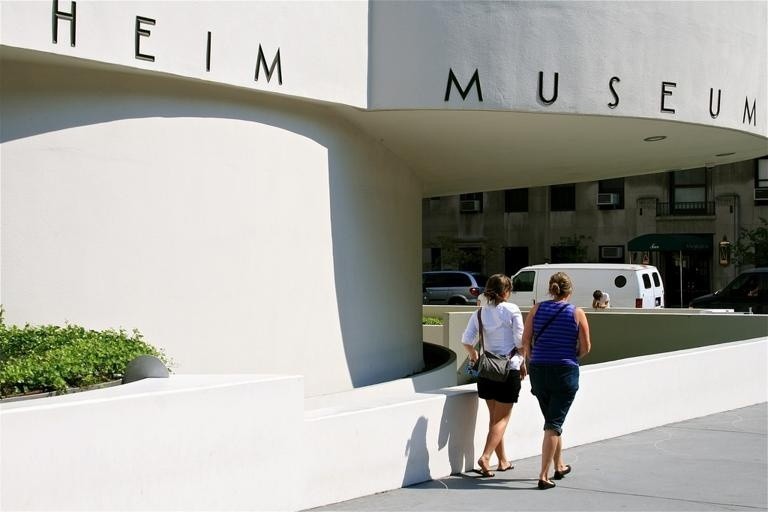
[747,279,758,296]
[460,273,529,477]
[592,290,611,308]
[521,271,592,491]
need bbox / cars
[423,271,485,305]
[689,268,768,314]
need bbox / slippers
[471,468,495,478]
[497,463,514,471]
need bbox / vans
[477,263,664,309]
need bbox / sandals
[538,479,555,490]
[554,465,571,480]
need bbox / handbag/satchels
[478,350,509,382]
